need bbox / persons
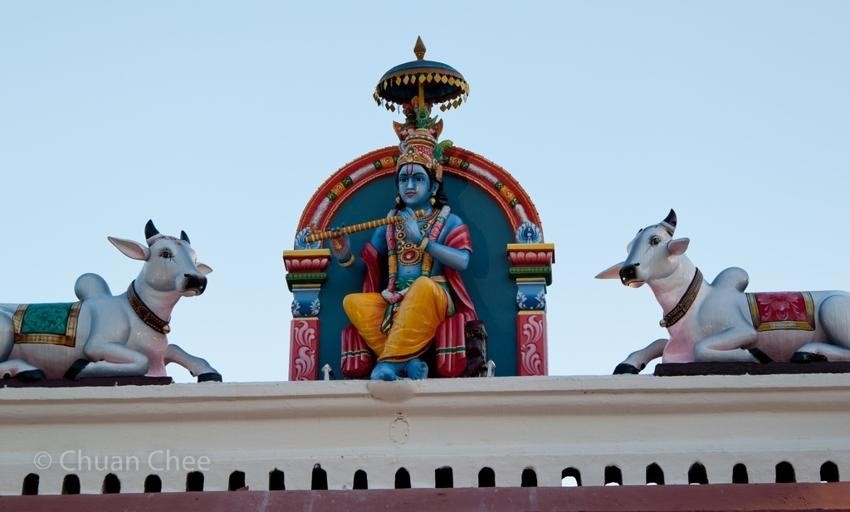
[326,127,478,380]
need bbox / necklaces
[395,209,441,266]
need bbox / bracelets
[414,235,429,251]
[338,254,355,267]
